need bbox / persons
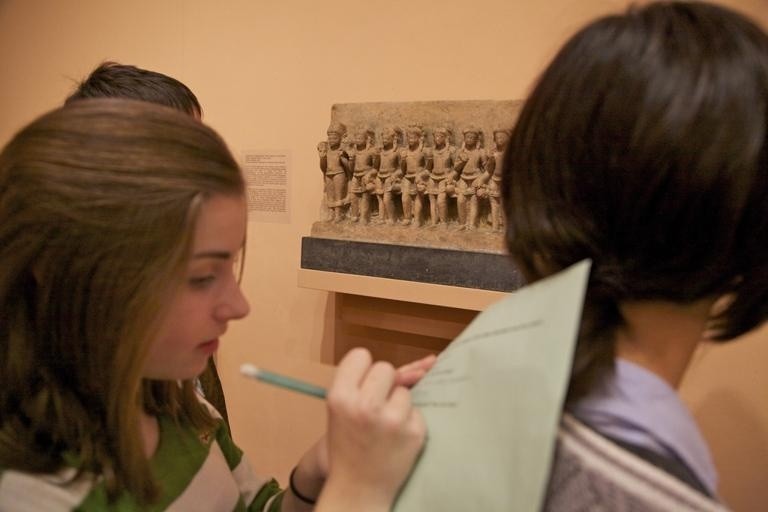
[65,61,231,436]
[499,0,768,512]
[317,121,511,235]
[0,97,440,511]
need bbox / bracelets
[288,465,316,504]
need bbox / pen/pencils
[240,363,327,399]
